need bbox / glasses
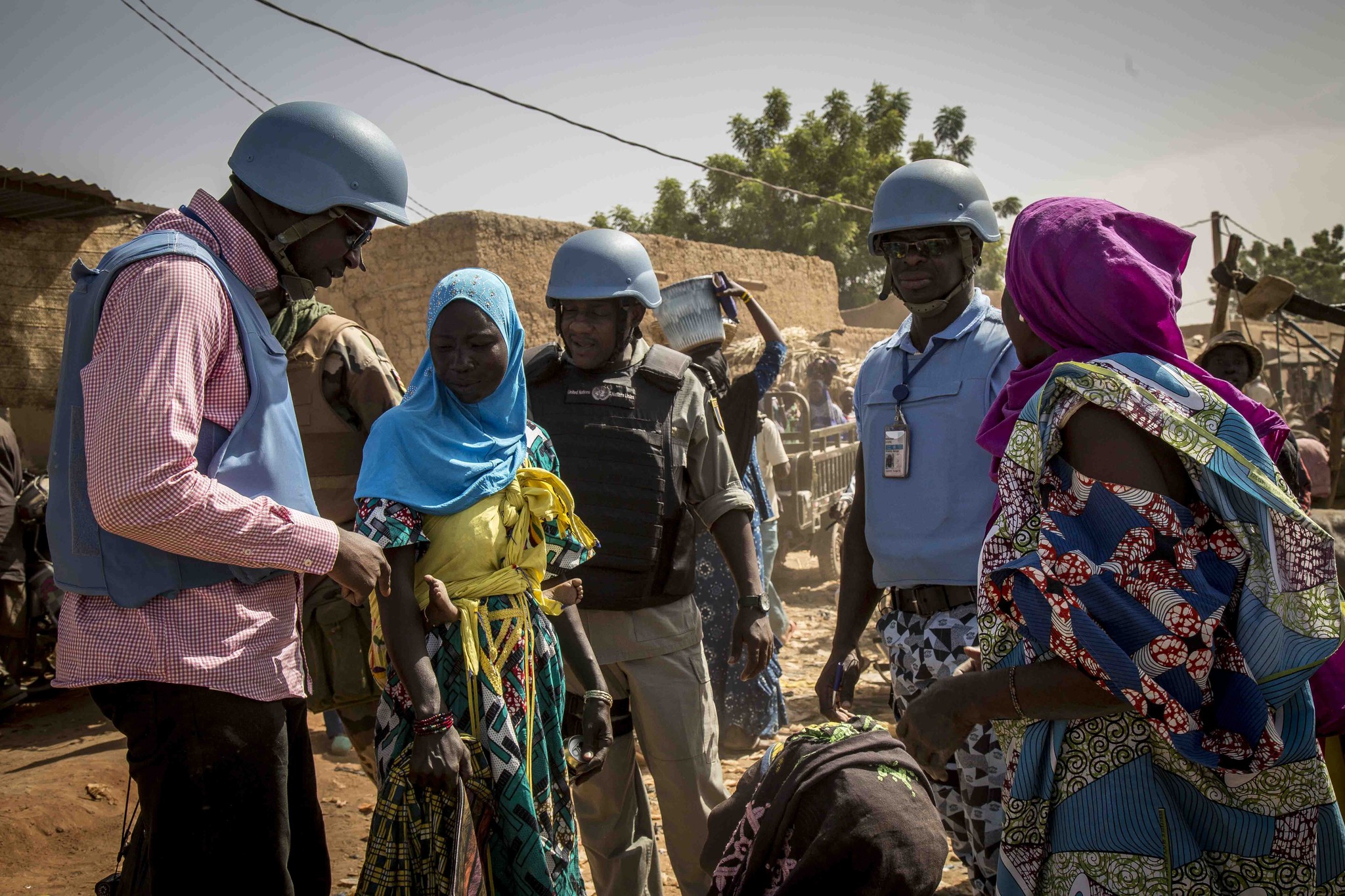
[331,205,371,250]
[880,236,959,261]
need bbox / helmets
[866,157,1001,255]
[227,100,410,229]
[545,228,663,311]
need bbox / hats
[1196,329,1264,384]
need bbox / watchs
[736,592,771,613]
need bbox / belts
[888,583,981,617]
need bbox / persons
[43,101,416,896]
[752,408,796,645]
[521,228,775,896]
[894,195,1345,896]
[1189,324,1264,395]
[264,294,406,786]
[699,713,950,896]
[323,708,353,756]
[0,413,31,718]
[354,267,613,894]
[829,471,857,520]
[647,269,790,752]
[811,155,1021,896]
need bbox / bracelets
[741,291,752,303]
[1009,664,1026,719]
[414,712,454,736]
[583,690,613,708]
